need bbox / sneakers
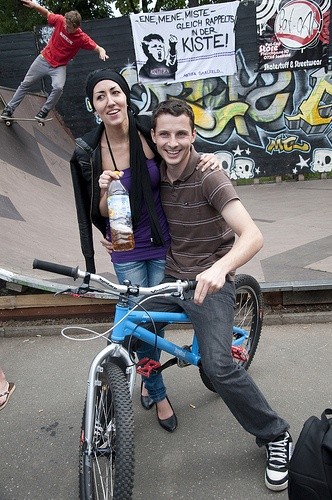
[0,110,12,119]
[35,110,48,120]
[263,431,293,492]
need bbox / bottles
[107,171,134,253]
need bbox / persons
[100,100,295,492]
[0,368,15,410]
[1,0,109,119]
[71,70,222,432]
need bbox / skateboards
[1,115,55,127]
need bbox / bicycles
[33,258,264,500]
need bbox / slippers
[0,382,17,411]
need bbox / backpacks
[287,408,332,500]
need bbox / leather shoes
[155,395,178,432]
[140,382,155,410]
